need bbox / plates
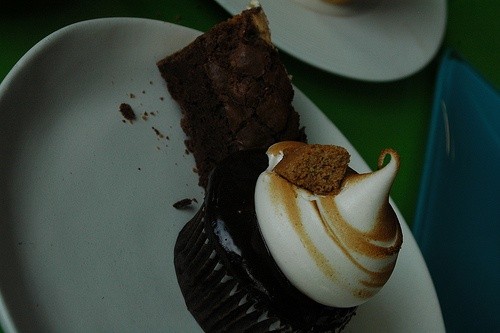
[1,17,447,333]
[412,55,499,332]
[216,0,448,84]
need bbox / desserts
[174,141,402,333]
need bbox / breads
[157,0,310,192]
